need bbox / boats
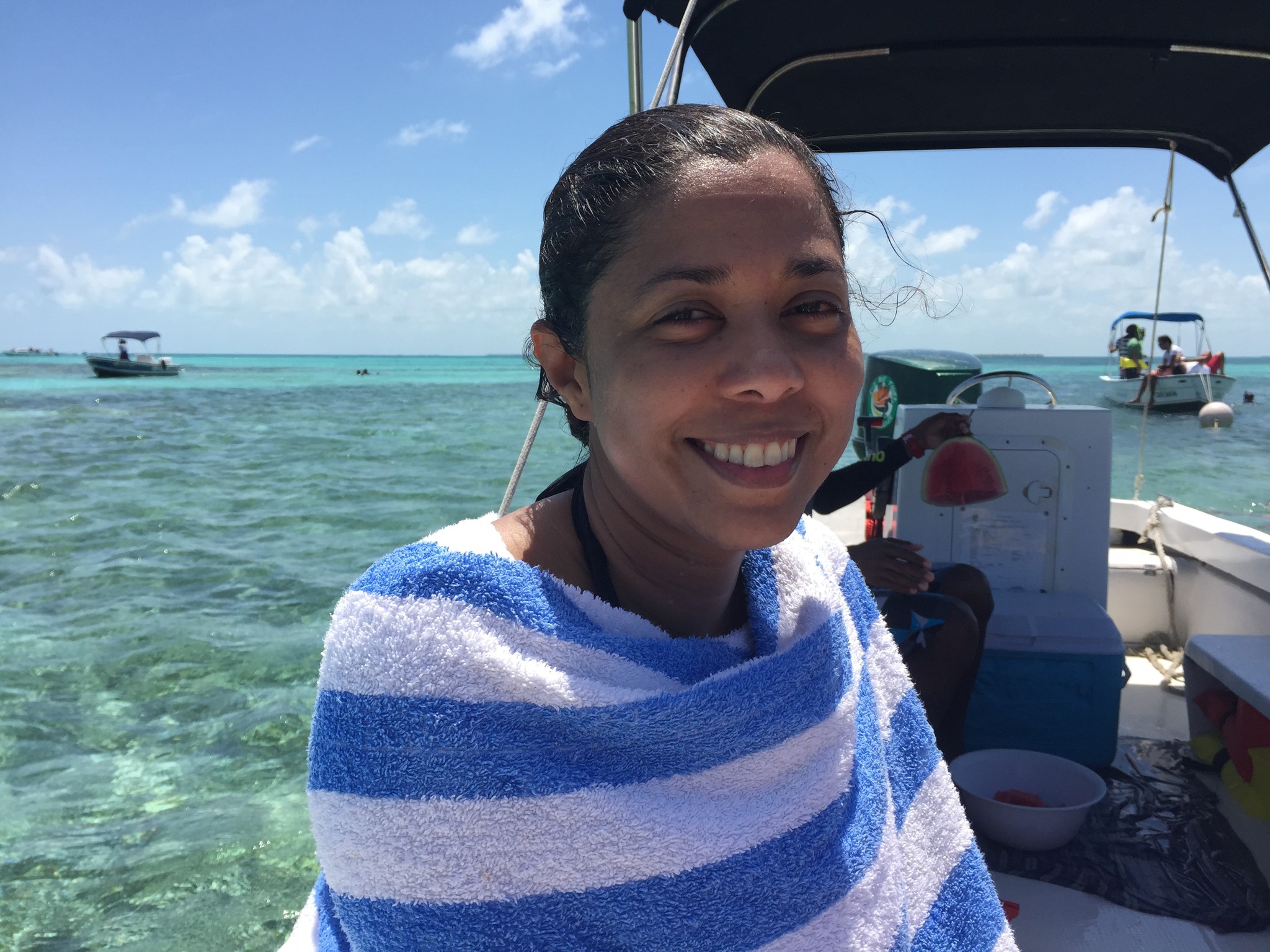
[1099,310,1237,416]
[1,348,59,356]
[84,330,182,378]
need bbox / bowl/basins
[949,749,1107,852]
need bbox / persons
[1170,350,1225,375]
[1109,324,1149,379]
[804,412,996,763]
[1128,335,1185,404]
[307,104,1018,951]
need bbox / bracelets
[901,431,925,459]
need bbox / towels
[306,508,1025,952]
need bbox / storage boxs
[964,587,1130,770]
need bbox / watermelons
[997,790,1051,808]
[920,435,1009,506]
